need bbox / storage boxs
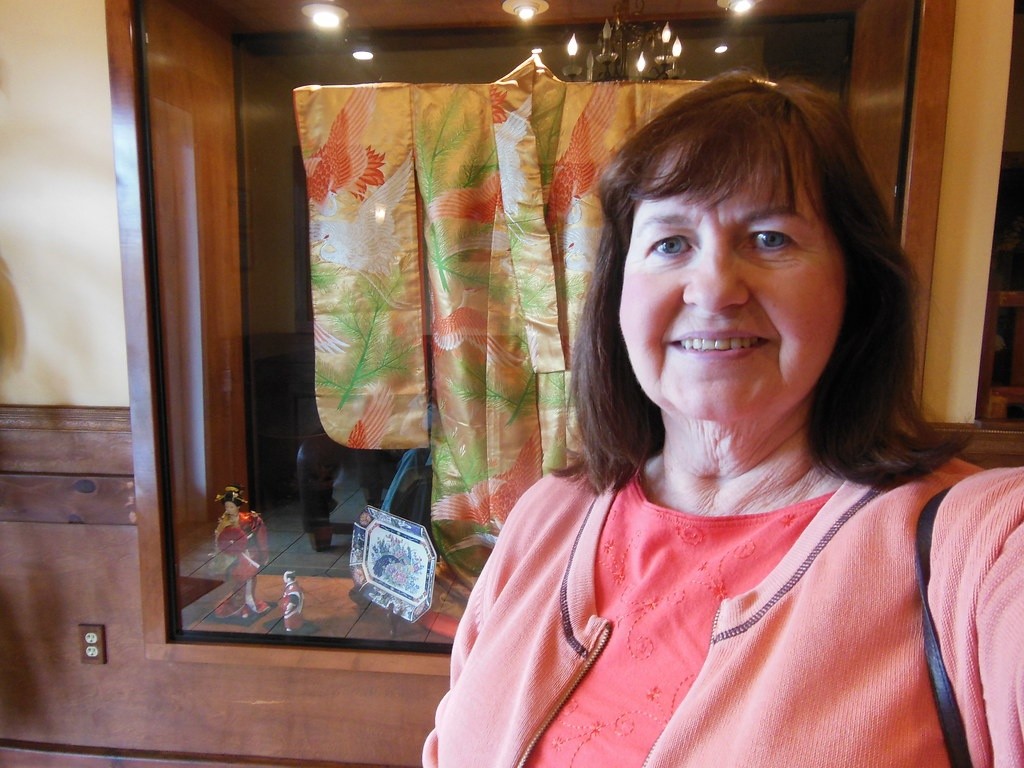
[78,623,107,665]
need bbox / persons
[280,570,306,631]
[208,485,271,619]
[422,72,1024,768]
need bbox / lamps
[561,0,686,82]
